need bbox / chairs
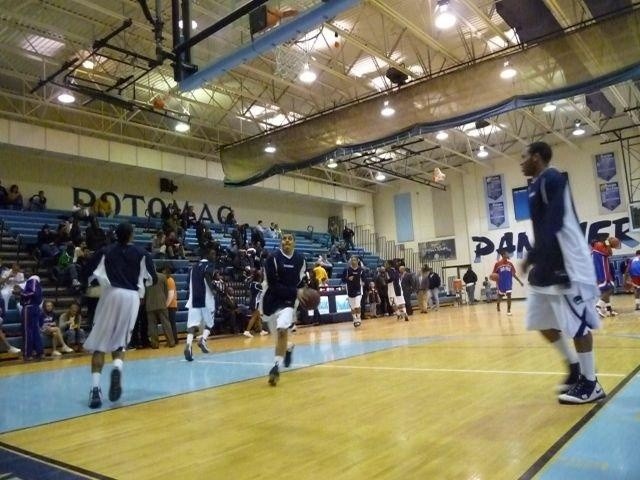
[0,201,459,359]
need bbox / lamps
[430,0,587,185]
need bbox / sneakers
[268,365,279,385]
[284,351,291,368]
[197,337,209,352]
[109,367,122,402]
[87,387,103,409]
[559,373,607,405]
[184,344,193,361]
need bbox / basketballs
[299,289,320,311]
[609,238,619,248]
[491,272,499,281]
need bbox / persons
[255,231,308,387]
[463,266,478,305]
[591,239,640,318]
[0,185,442,363]
[83,221,158,408]
[490,248,524,316]
[520,143,606,405]
[483,276,490,303]
